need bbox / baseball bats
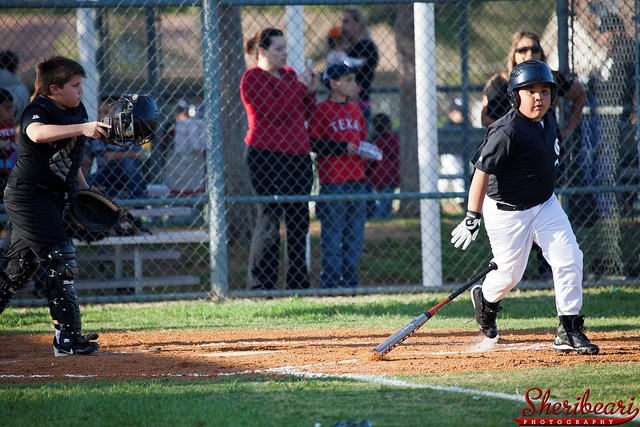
[368,262,498,360]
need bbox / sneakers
[552,314,599,354]
[52,336,99,356]
[470,284,503,338]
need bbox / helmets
[99,92,159,150]
[506,59,558,108]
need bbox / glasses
[515,46,541,53]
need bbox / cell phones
[308,58,313,69]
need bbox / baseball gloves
[68,188,127,234]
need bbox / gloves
[450,209,482,250]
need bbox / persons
[451,60,599,354]
[341,7,381,104]
[166,100,208,194]
[310,64,384,290]
[596,13,638,124]
[480,31,590,135]
[0,88,16,174]
[0,55,114,358]
[371,112,399,221]
[95,96,152,200]
[324,27,368,72]
[240,34,319,289]
[4,48,28,109]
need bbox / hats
[320,61,363,86]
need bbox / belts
[496,202,534,211]
